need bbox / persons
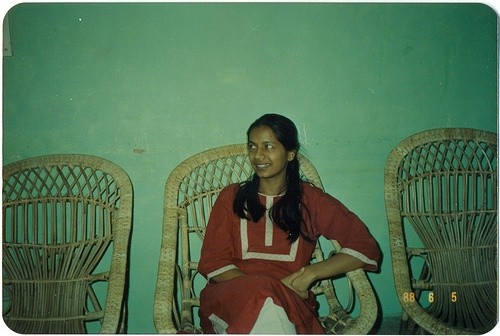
[194,111,384,336]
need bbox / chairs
[381,127,499,335]
[150,143,380,334]
[2,152,134,334]
